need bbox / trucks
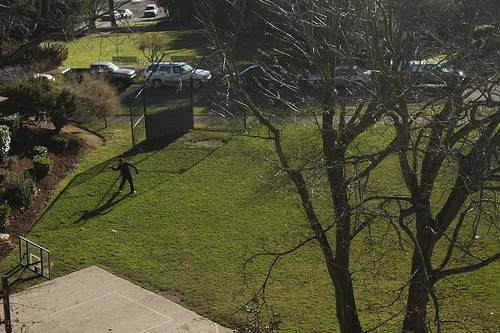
[305,65,387,91]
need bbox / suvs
[145,62,212,89]
[397,59,469,94]
[144,3,158,17]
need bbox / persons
[110,158,139,193]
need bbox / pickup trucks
[62,62,136,88]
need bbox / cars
[117,9,133,19]
[224,62,304,91]
[101,9,121,20]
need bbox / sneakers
[119,189,122,193]
[131,191,136,193]
